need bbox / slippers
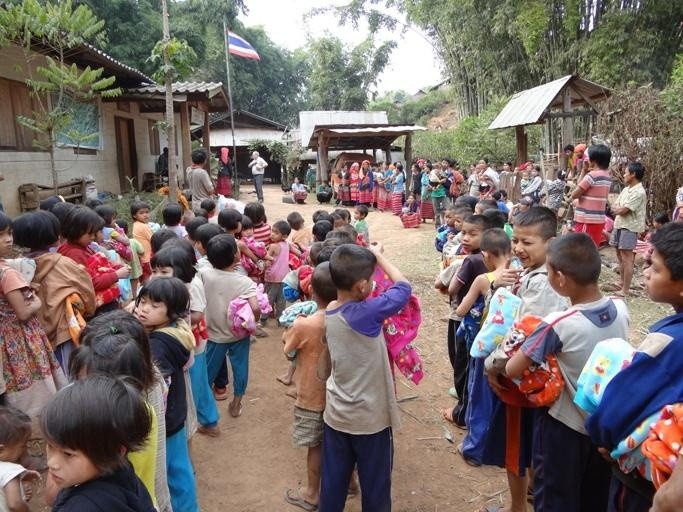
[198,424,218,437]
[230,401,243,417]
[213,385,228,400]
[285,488,316,511]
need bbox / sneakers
[444,407,467,430]
[260,318,267,326]
[449,385,458,398]
[252,324,268,337]
[17,437,49,472]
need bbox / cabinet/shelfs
[143,172,163,192]
[17,177,88,213]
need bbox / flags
[226,28,263,62]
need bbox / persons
[1,141,683,512]
